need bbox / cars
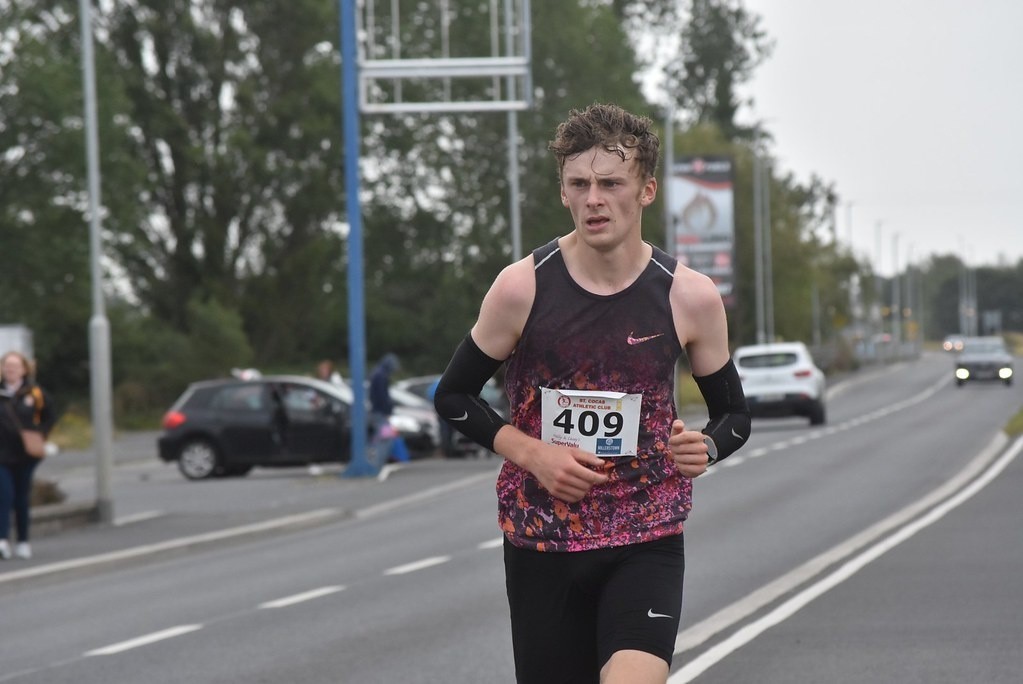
[155,373,513,483]
[944,333,965,356]
[952,335,1015,387]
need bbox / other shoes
[16,541,32,558]
[0,539,11,558]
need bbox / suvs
[732,340,829,426]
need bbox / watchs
[704,437,717,464]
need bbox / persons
[431,104,751,684]
[318,361,352,399]
[0,351,54,560]
[369,353,401,464]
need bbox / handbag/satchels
[21,428,46,456]
